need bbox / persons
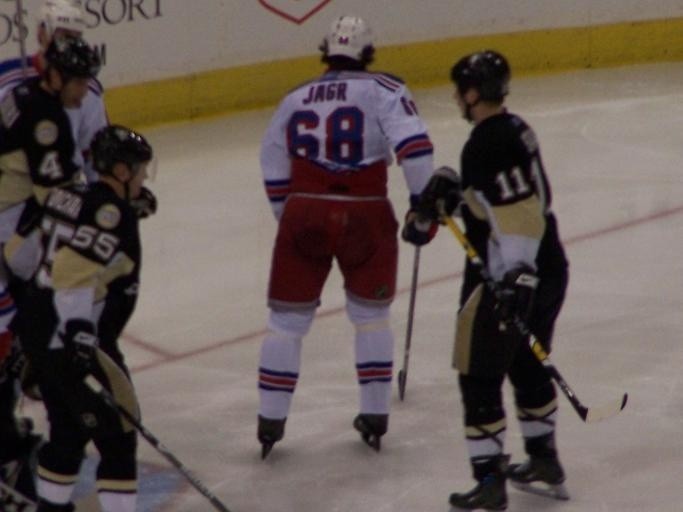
[449,50,568,512]
[258,14,440,443]
[0,0,157,512]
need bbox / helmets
[43,0,101,81]
[323,16,374,68]
[449,49,512,100]
[85,124,154,176]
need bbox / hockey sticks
[398,242,423,403]
[437,198,630,426]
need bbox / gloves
[63,318,103,376]
[130,186,157,218]
[400,167,461,246]
[491,263,538,322]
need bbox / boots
[353,413,389,435]
[448,454,511,510]
[257,414,287,444]
[507,430,567,485]
